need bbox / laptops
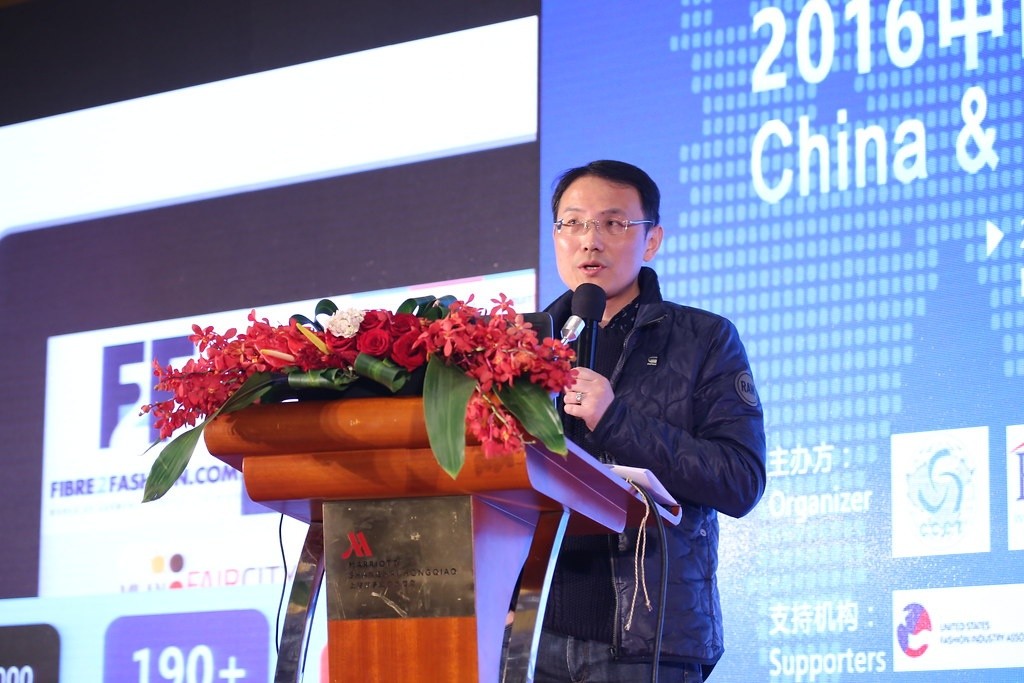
[467,311,553,346]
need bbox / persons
[500,160,766,683]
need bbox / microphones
[561,316,585,346]
[571,283,606,370]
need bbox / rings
[576,391,583,402]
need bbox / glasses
[553,216,655,237]
